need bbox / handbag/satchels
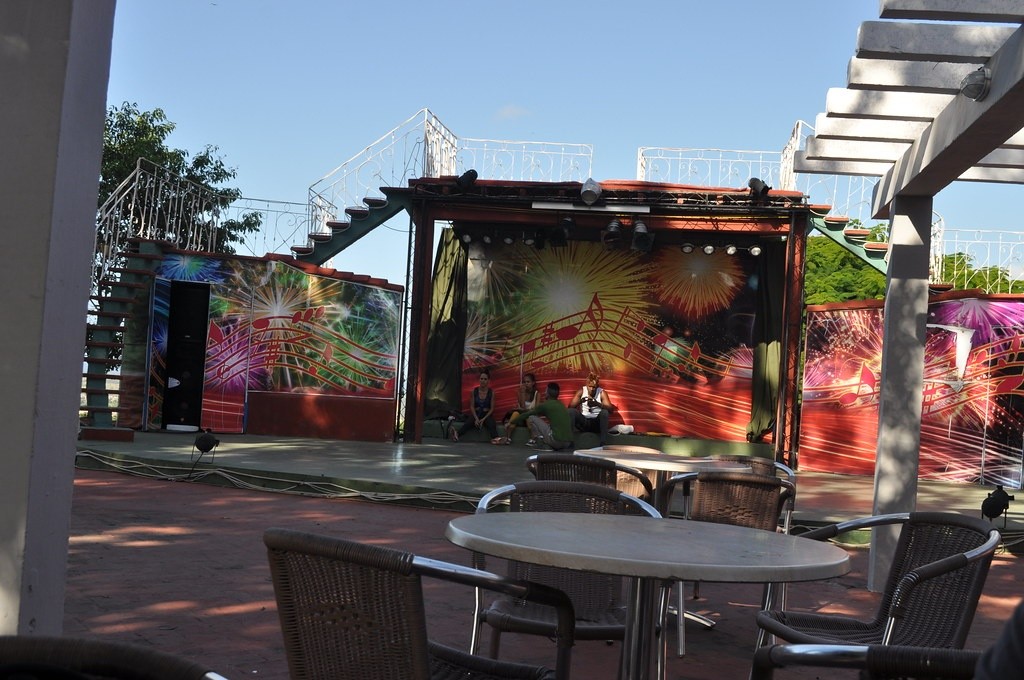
[425,411,451,420]
[608,424,635,435]
[453,409,469,422]
[502,409,523,424]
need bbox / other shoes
[506,436,512,445]
[568,441,575,449]
[490,437,507,447]
[599,441,608,447]
[536,444,554,451]
[448,425,460,442]
[524,438,538,446]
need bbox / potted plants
[864,223,890,259]
[844,218,872,245]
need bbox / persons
[504,383,573,451]
[567,371,613,451]
[502,374,541,446]
[447,370,508,444]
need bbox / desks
[444,511,852,680]
[573,449,752,633]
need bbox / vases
[824,216,849,231]
[810,204,832,219]
[929,284,954,295]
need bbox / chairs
[752,600,1024,680]
[262,527,575,680]
[682,454,797,600]
[591,443,679,514]
[637,471,796,659]
[0,634,228,680]
[525,454,653,504]
[748,512,1001,680]
[468,480,668,680]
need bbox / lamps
[503,234,515,245]
[195,434,216,453]
[748,245,763,257]
[681,243,695,254]
[748,177,772,196]
[453,169,478,193]
[982,484,1015,518]
[525,236,535,247]
[601,221,626,250]
[725,245,738,256]
[702,244,715,255]
[959,67,992,102]
[461,232,474,244]
[630,220,656,252]
[580,179,601,205]
[481,233,495,245]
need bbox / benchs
[423,420,775,459]
